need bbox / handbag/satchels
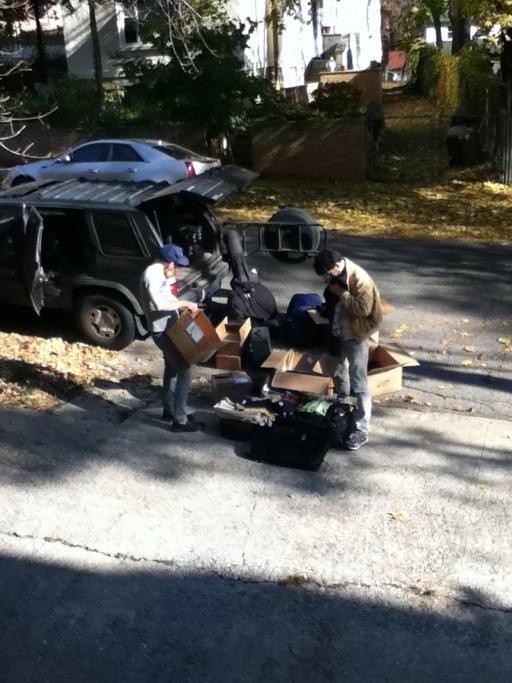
[292,396,365,448]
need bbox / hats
[160,243,190,266]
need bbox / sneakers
[346,430,369,450]
[163,413,207,432]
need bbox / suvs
[0,162,260,350]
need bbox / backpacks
[230,281,277,325]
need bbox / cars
[2,135,222,186]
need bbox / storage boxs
[166,304,420,405]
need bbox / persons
[314,248,380,450]
[140,244,206,433]
